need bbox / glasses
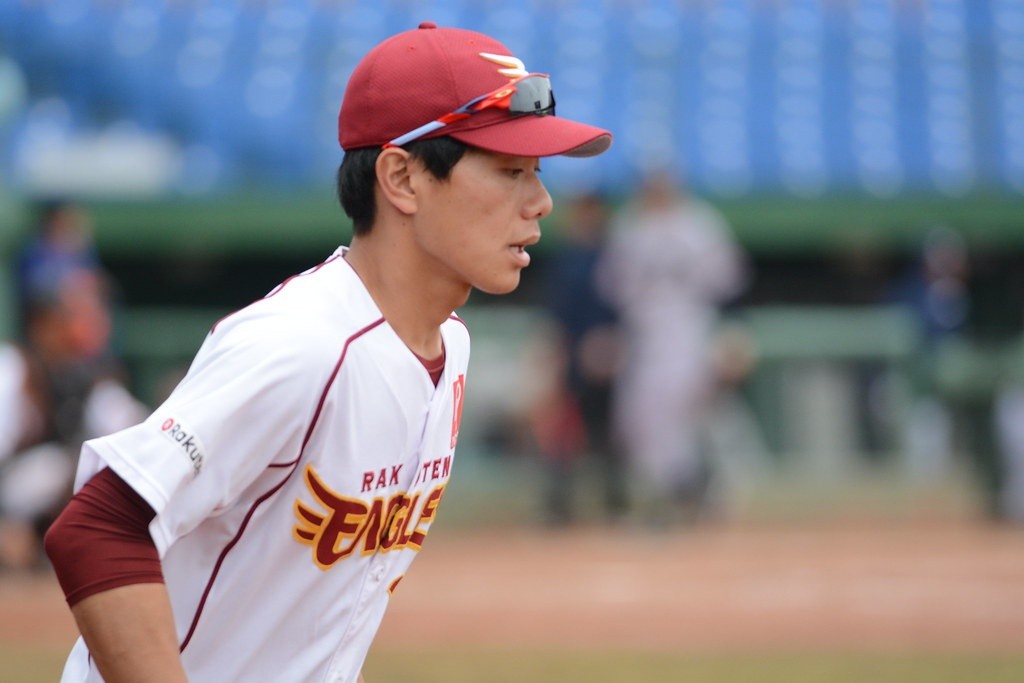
[377,72,559,150]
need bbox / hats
[336,20,612,161]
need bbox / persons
[43,22,612,683]
[15,194,121,434]
[549,148,746,525]
